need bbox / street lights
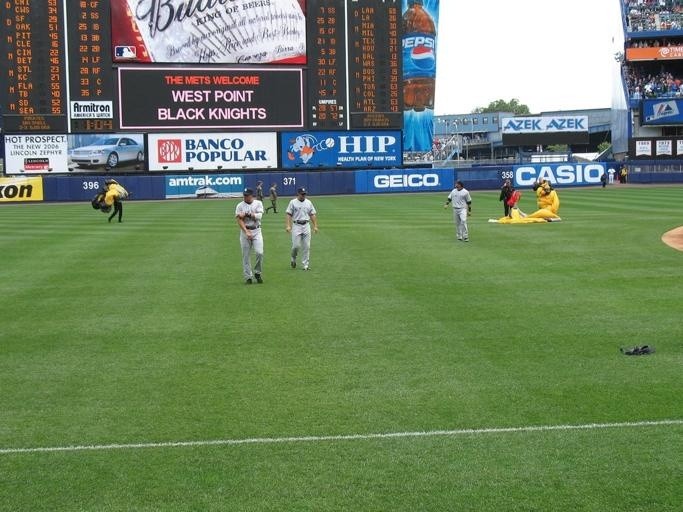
[438,116,497,171]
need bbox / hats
[243,188,253,196]
[297,188,307,195]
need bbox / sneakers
[291,256,296,268]
[465,238,469,242]
[255,273,263,283]
[303,268,311,271]
[247,279,252,284]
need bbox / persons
[235,187,265,283]
[499,179,515,216]
[265,183,277,213]
[601,174,607,187]
[608,167,616,184]
[108,196,122,222]
[621,0,683,97]
[286,187,318,270]
[534,177,553,195]
[619,167,623,183]
[622,167,627,183]
[255,181,264,201]
[402,133,484,161]
[444,180,471,242]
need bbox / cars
[71,134,143,167]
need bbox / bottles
[401,0,437,112]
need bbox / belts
[293,220,307,224]
[246,226,260,229]
